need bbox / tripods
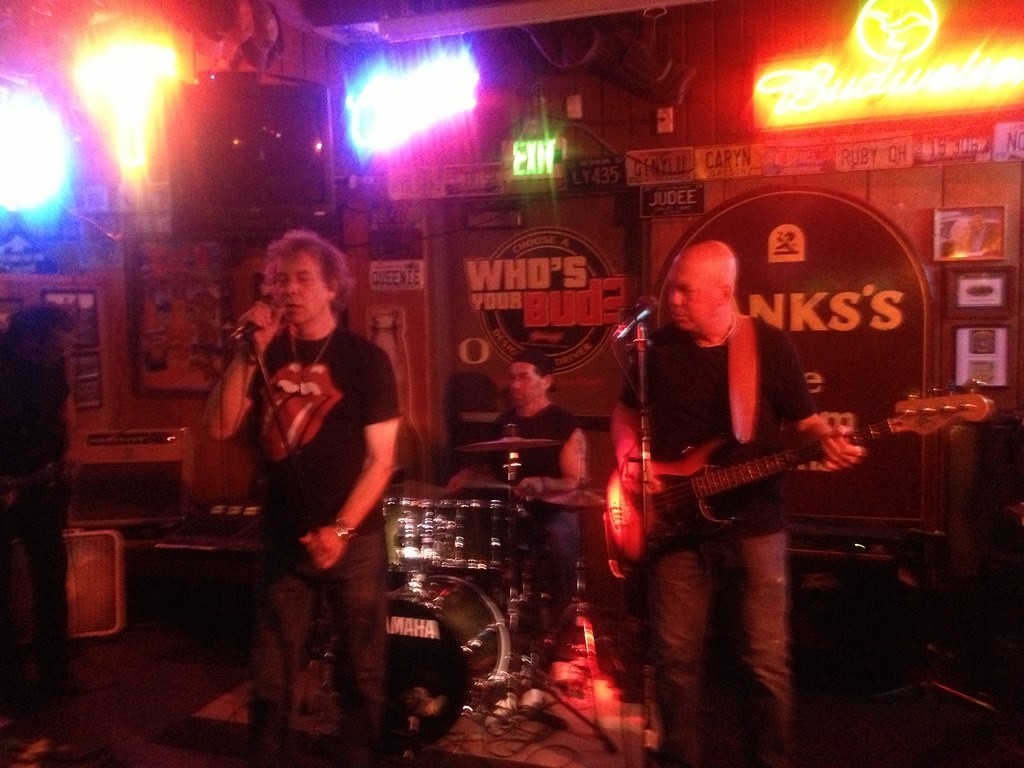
[478,451,642,757]
[870,544,997,713]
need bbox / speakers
[66,426,196,531]
[8,530,125,644]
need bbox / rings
[857,446,862,456]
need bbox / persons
[611,241,869,768]
[202,229,401,768]
[0,304,90,722]
[444,350,587,625]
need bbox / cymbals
[456,436,562,454]
[539,487,607,507]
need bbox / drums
[327,575,511,751]
[396,496,511,571]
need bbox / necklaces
[288,322,337,382]
[696,309,736,346]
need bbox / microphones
[226,293,280,344]
[908,527,944,544]
[612,295,658,340]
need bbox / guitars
[605,378,998,576]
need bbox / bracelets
[331,518,358,542]
